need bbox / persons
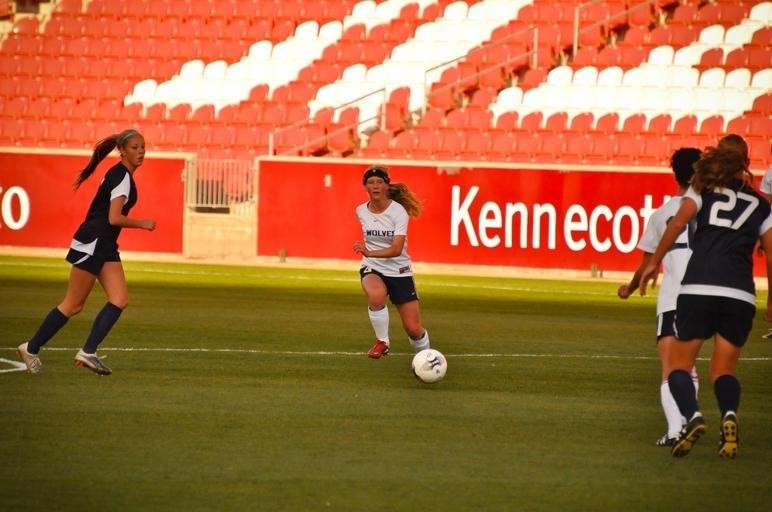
[618,147,700,446]
[639,134,772,460]
[352,165,430,359]
[17,129,156,377]
[758,167,772,339]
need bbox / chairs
[1,2,772,178]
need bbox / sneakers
[71,345,115,378]
[654,430,681,447]
[715,408,743,461]
[366,338,392,361]
[15,340,48,376]
[669,415,709,458]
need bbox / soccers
[412,349,447,384]
